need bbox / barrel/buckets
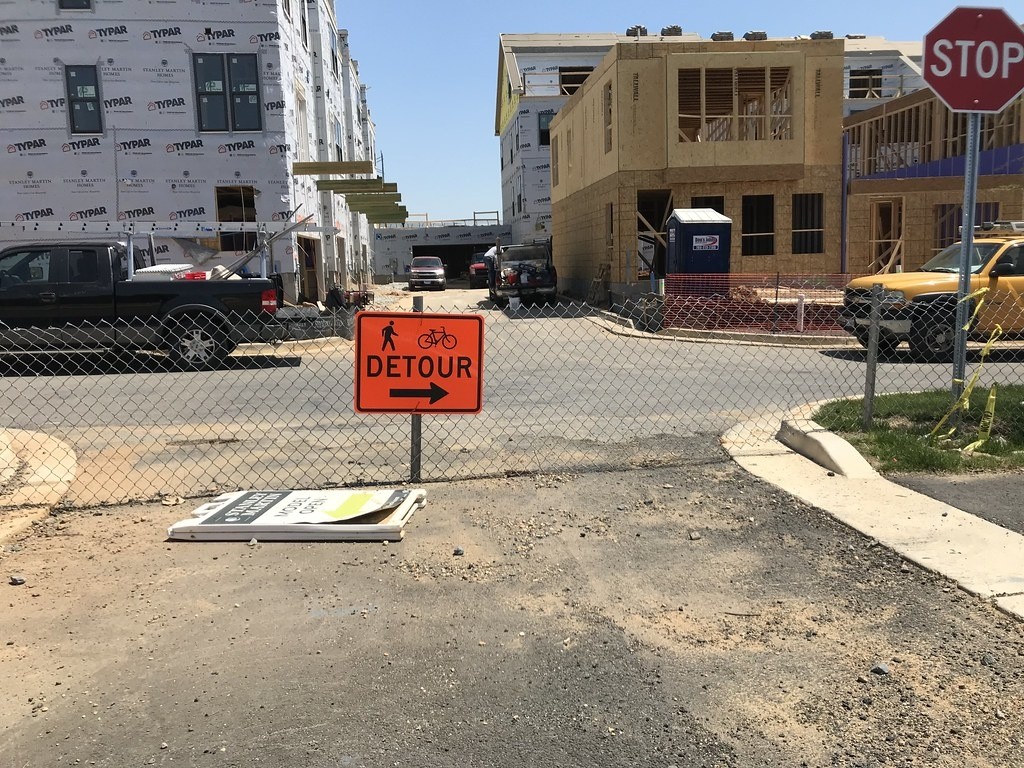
[509,297,520,310]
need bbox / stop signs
[920,6,1024,113]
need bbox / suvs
[469,253,489,289]
[406,257,447,291]
[837,219,1024,362]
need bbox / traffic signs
[354,311,484,415]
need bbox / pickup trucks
[489,246,558,308]
[0,241,284,372]
